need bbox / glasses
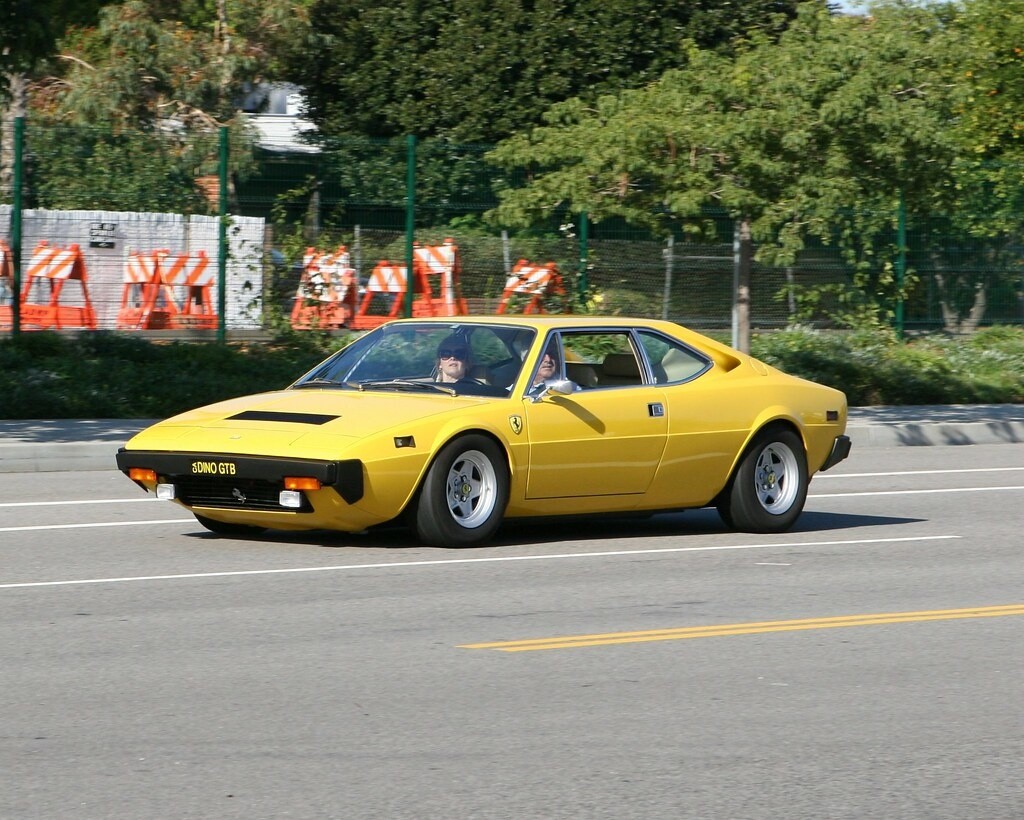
[439,349,468,360]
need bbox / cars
[113,314,854,548]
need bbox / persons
[505,330,581,399]
[435,336,473,383]
